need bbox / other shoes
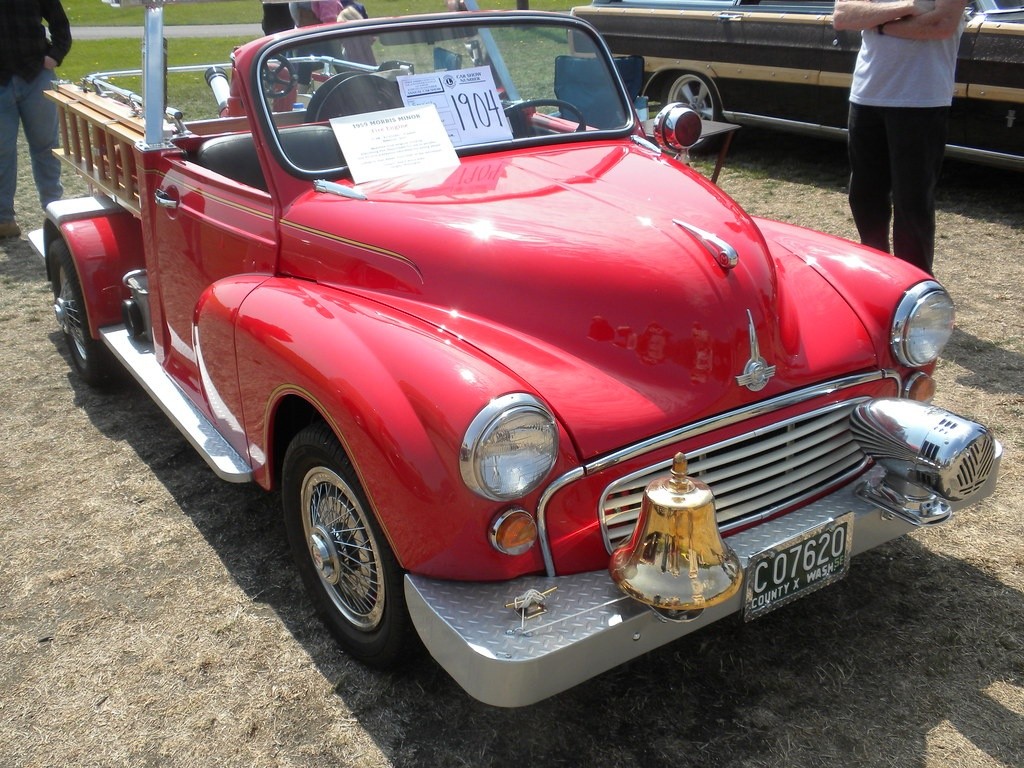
[0,220,21,238]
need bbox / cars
[568,0,1024,186]
[29,0,1004,710]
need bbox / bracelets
[878,23,886,35]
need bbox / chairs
[195,125,354,193]
[554,53,646,128]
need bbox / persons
[831,1,969,275]
[1,1,72,243]
[288,0,376,94]
